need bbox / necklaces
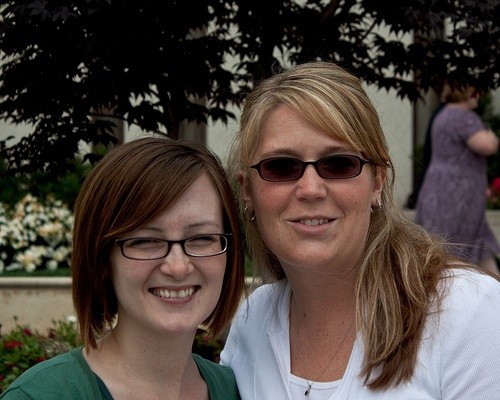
[288,293,353,396]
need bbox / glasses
[109,232,234,261]
[249,154,371,183]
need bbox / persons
[0,136,243,400]
[413,76,500,282]
[218,62,500,400]
